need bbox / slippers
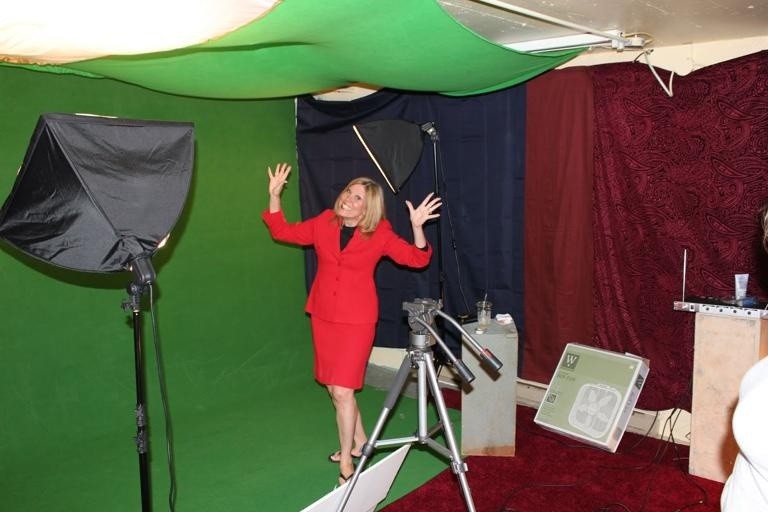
[329,453,360,463]
[340,464,356,482]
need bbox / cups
[477,300,493,327]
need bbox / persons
[719,203,768,511]
[268,161,443,486]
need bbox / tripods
[336,357,477,512]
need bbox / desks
[462,318,519,457]
[688,312,767,484]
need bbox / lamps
[352,116,445,435]
[0,112,195,512]
[504,29,622,53]
